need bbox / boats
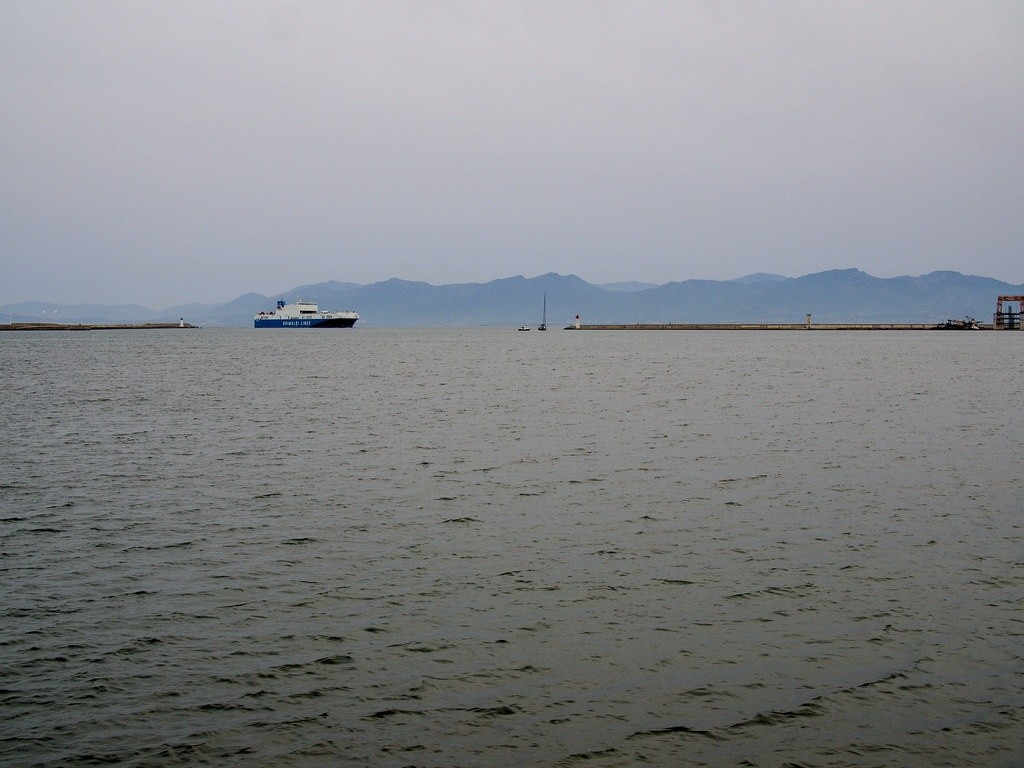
[253,298,360,328]
